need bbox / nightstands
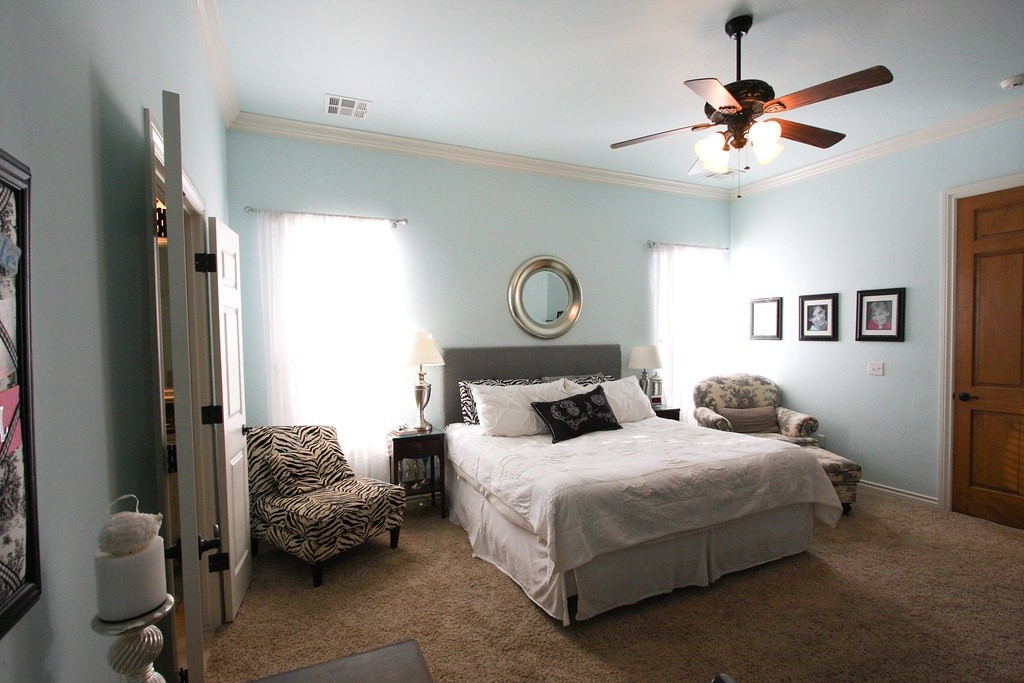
[381,428,448,518]
[654,405,681,421]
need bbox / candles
[94,536,167,621]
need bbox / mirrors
[508,255,584,341]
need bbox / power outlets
[816,433,827,449]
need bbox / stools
[802,444,863,515]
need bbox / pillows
[564,376,655,426]
[547,372,613,388]
[714,405,780,434]
[267,440,351,495]
[531,388,619,444]
[467,379,570,436]
[458,377,543,424]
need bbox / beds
[428,341,843,626]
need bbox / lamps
[398,332,445,430]
[629,345,665,401]
[695,119,785,177]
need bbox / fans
[610,14,893,174]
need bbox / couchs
[243,424,405,587]
[693,374,821,448]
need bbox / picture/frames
[0,150,42,640]
[854,286,910,343]
[797,293,839,340]
[750,295,782,340]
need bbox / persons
[866,302,890,330]
[808,305,827,330]
